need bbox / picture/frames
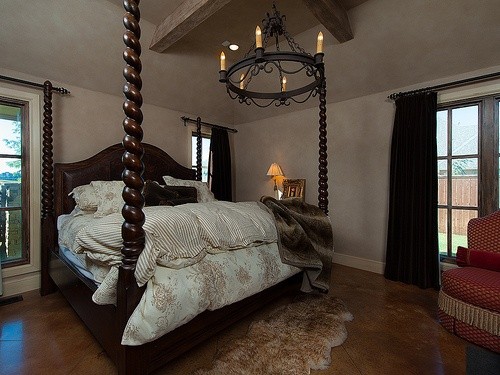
[282,178,305,202]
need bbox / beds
[41,0,335,375]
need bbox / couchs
[436,209,500,354]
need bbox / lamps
[219,0,326,108]
[267,162,284,200]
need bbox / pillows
[66,176,216,216]
[457,246,500,273]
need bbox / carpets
[465,343,500,375]
[192,291,352,375]
